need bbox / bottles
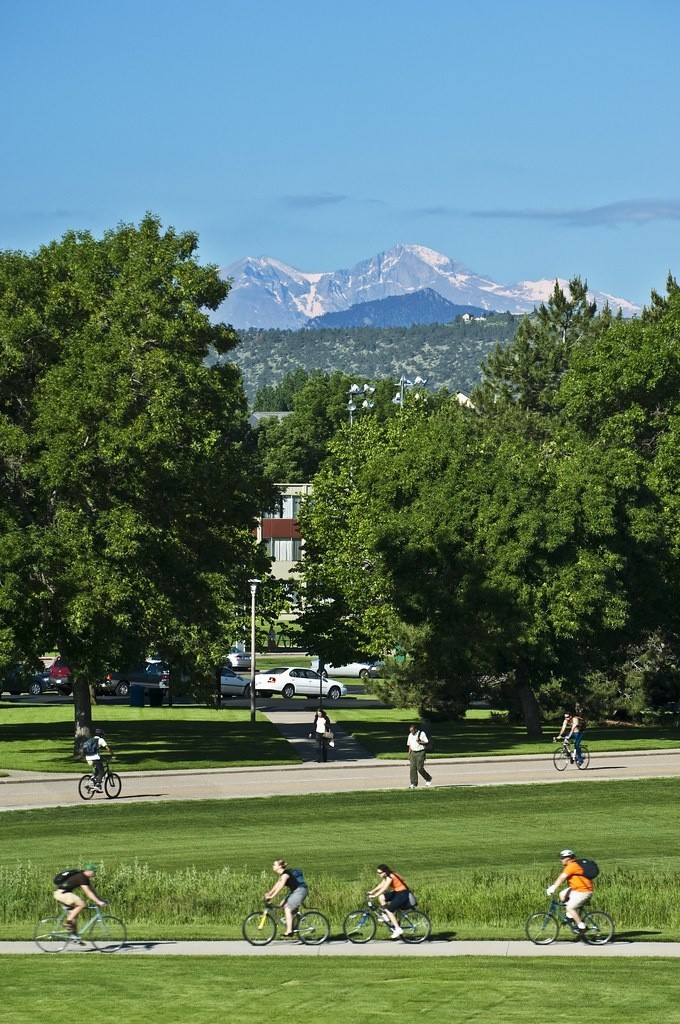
[280,917,286,924]
[381,911,389,922]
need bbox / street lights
[247,578,262,723]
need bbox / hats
[95,729,104,735]
[85,864,98,872]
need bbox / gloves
[546,885,556,893]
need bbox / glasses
[565,716,570,718]
[560,857,565,860]
[376,872,383,874]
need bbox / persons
[53,864,106,945]
[308,708,331,762]
[546,849,595,930]
[84,729,115,793]
[556,712,585,766]
[406,725,432,788]
[367,864,416,938]
[264,858,307,937]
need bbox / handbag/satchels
[323,729,333,738]
[401,890,416,910]
[329,738,334,747]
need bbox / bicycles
[526,892,615,946]
[78,756,122,800]
[32,899,128,953]
[241,899,330,946]
[342,891,432,944]
[552,736,590,771]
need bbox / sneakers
[391,927,404,939]
[378,914,390,922]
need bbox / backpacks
[82,737,103,756]
[418,728,434,753]
[53,870,81,885]
[572,859,599,880]
[573,716,587,732]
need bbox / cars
[213,667,252,699]
[96,658,170,696]
[310,656,382,679]
[0,656,73,696]
[249,667,347,700]
[221,646,257,671]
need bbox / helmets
[559,850,574,856]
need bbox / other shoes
[63,923,86,946]
[579,925,589,937]
[565,752,584,767]
[280,933,293,937]
[89,776,103,792]
[425,781,431,786]
[561,917,574,926]
[409,784,417,789]
